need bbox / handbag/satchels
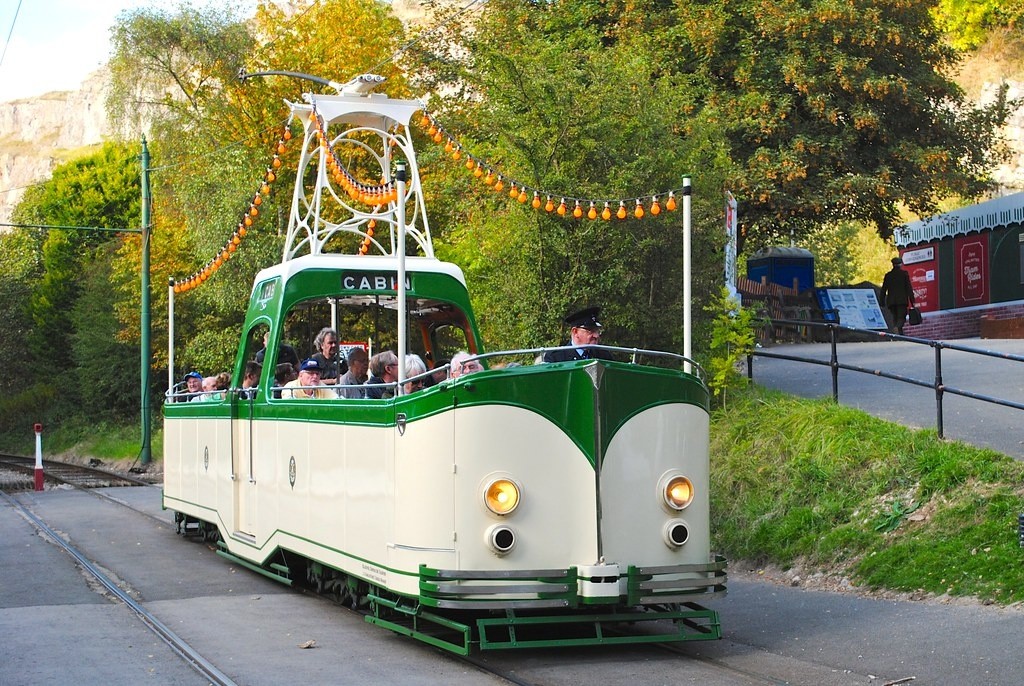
[908,305,922,325]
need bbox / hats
[891,258,902,264]
[565,306,602,327]
[300,357,320,370]
[184,371,202,381]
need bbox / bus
[157,66,729,653]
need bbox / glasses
[577,327,602,335]
[304,369,320,375]
[412,379,427,385]
[288,367,296,375]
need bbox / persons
[254,331,302,376]
[173,372,203,402]
[425,360,450,387]
[301,326,351,384]
[450,351,479,384]
[880,257,915,336]
[281,358,340,401]
[190,372,231,403]
[230,361,262,401]
[544,305,617,361]
[331,347,369,400]
[382,354,428,398]
[272,362,298,398]
[354,351,397,400]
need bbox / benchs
[737,291,840,347]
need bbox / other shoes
[893,327,900,335]
